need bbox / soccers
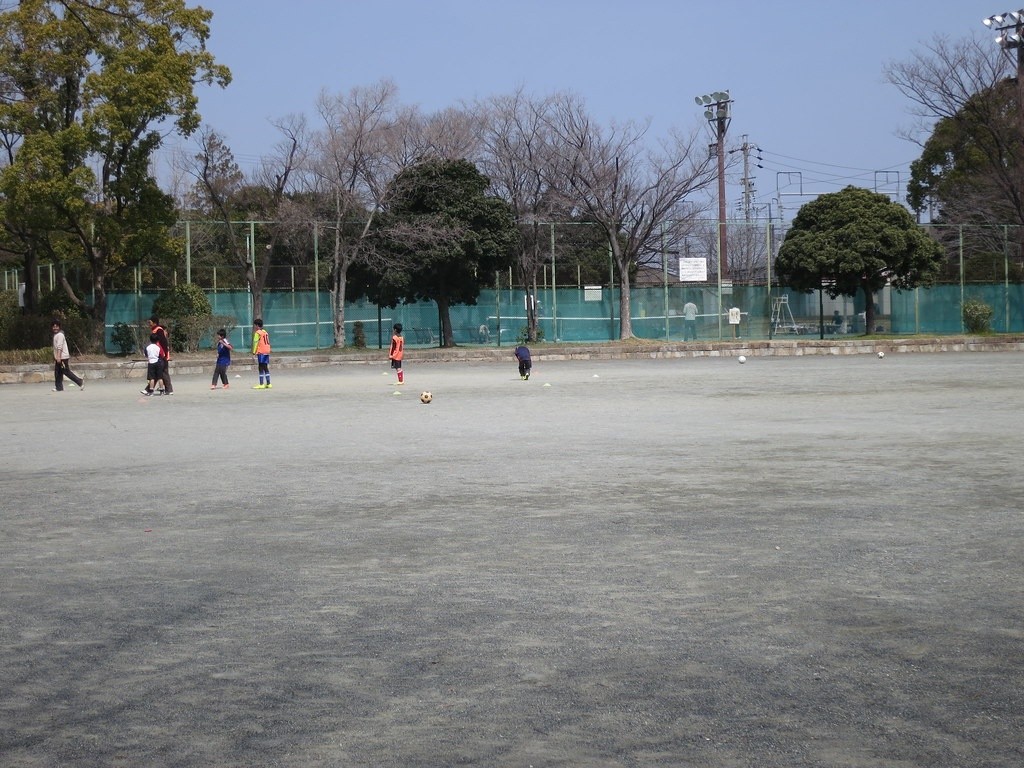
[877,351,885,359]
[420,391,433,403]
[738,356,746,364]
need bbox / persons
[732,302,741,339]
[140,315,173,396]
[210,329,232,389]
[251,318,272,389]
[681,297,698,342]
[389,323,404,385]
[515,346,531,380]
[52,321,86,392]
[479,324,490,344]
[832,311,843,335]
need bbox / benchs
[469,325,499,344]
[414,328,442,345]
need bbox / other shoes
[146,389,154,396]
[166,391,173,395]
[211,384,216,388]
[394,382,404,385]
[80,379,84,390]
[52,388,64,392]
[222,383,229,388]
[159,389,164,397]
[140,390,149,395]
[521,373,529,380]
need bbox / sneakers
[266,384,272,388]
[253,384,265,389]
